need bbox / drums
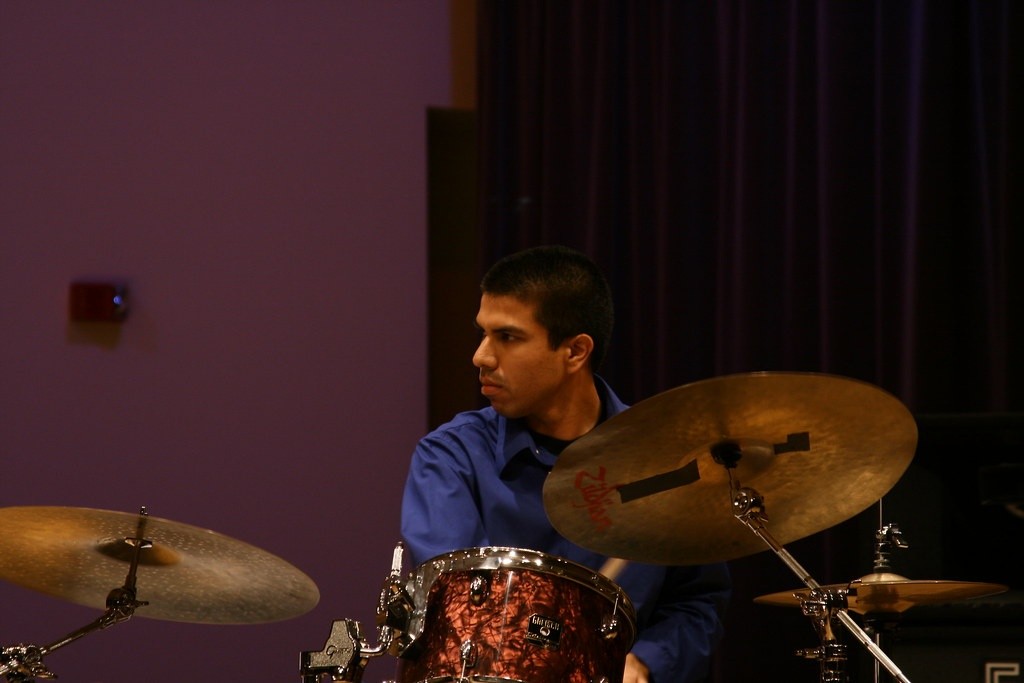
[392,546,639,683]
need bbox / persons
[400,244,754,683]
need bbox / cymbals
[1,505,322,627]
[544,371,920,564]
[752,570,1008,621]
